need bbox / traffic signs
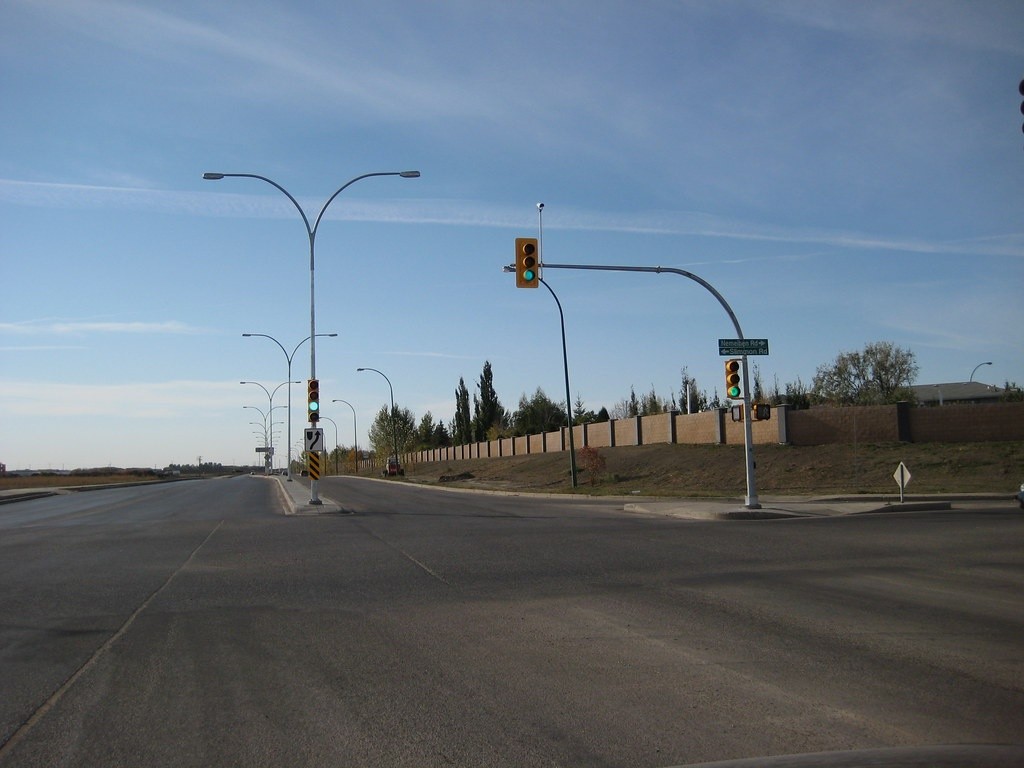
[718,339,768,355]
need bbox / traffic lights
[725,361,741,398]
[308,380,319,422]
[516,238,539,288]
[757,404,770,420]
[732,404,744,422]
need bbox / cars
[250,471,256,474]
[282,470,288,475]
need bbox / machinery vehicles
[383,457,404,477]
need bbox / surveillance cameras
[537,202,544,208]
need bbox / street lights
[502,266,577,489]
[243,334,337,483]
[320,417,338,476]
[243,406,289,476]
[357,368,398,477]
[285,438,308,471]
[239,381,301,475]
[333,400,358,476]
[203,172,420,506]
